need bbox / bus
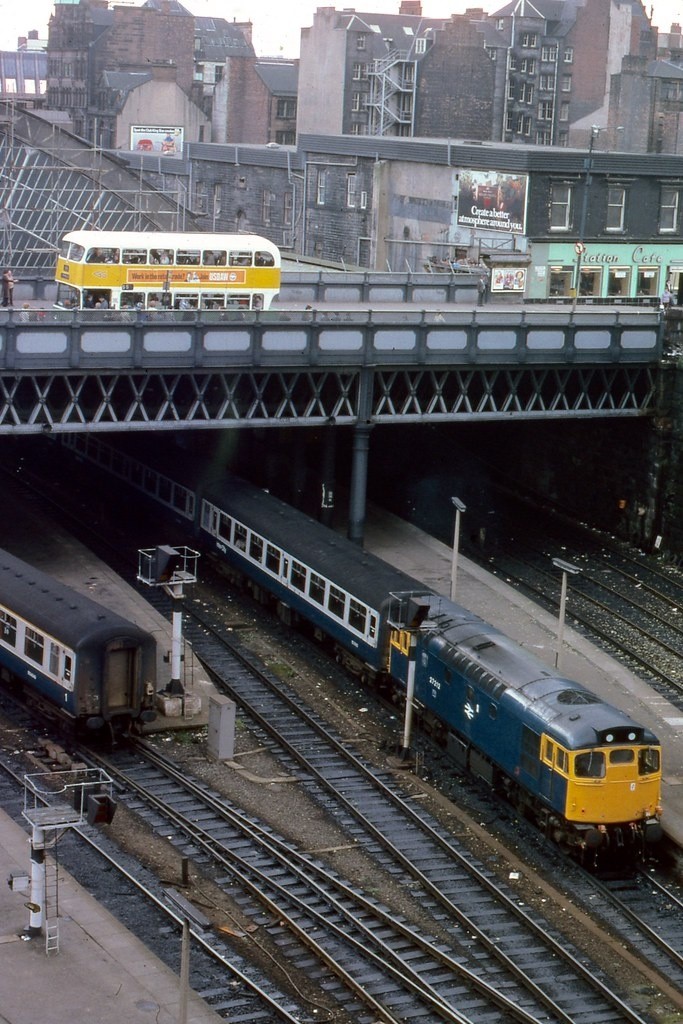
[51,230,282,311]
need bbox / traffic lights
[403,596,432,628]
[85,793,119,828]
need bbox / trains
[0,550,160,749]
[0,401,665,872]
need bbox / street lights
[448,495,468,603]
[574,125,628,295]
[552,556,581,669]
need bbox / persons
[661,279,674,310]
[19,303,46,321]
[476,276,485,306]
[431,256,472,268]
[0,270,18,307]
[434,310,445,322]
[63,245,263,321]
[280,305,352,322]
